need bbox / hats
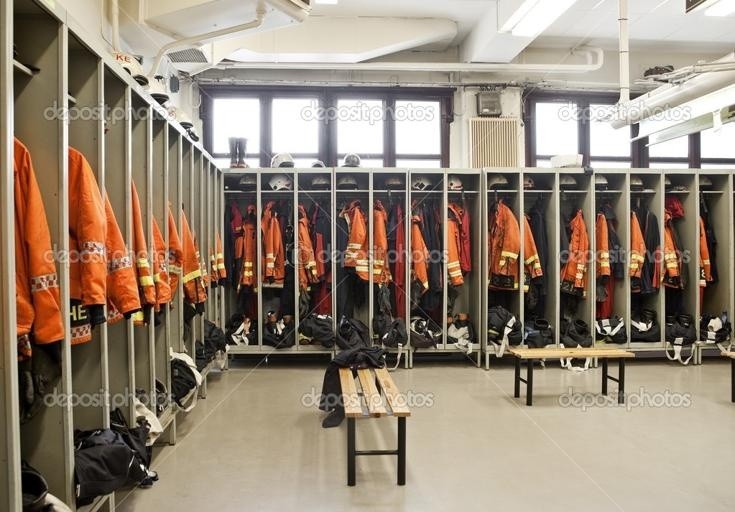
[311,159,326,167]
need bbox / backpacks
[447,312,477,344]
[382,317,408,348]
[336,312,371,351]
[23,320,225,510]
[262,311,295,347]
[524,318,555,348]
[488,305,523,346]
[631,309,660,342]
[701,310,731,346]
[560,315,592,349]
[665,313,697,345]
[299,313,335,348]
[595,313,627,344]
[410,315,443,348]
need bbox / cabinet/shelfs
[0,0,733,512]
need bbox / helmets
[166,103,194,130]
[267,173,293,191]
[141,76,170,106]
[700,175,714,190]
[630,175,645,191]
[112,52,150,86]
[238,175,256,190]
[270,152,295,168]
[448,175,464,191]
[560,174,579,190]
[665,175,674,191]
[488,174,510,190]
[337,174,358,189]
[383,175,404,190]
[595,174,610,190]
[341,153,362,167]
[311,175,330,190]
[411,175,433,191]
[524,175,535,189]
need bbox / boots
[228,137,238,168]
[236,138,247,168]
